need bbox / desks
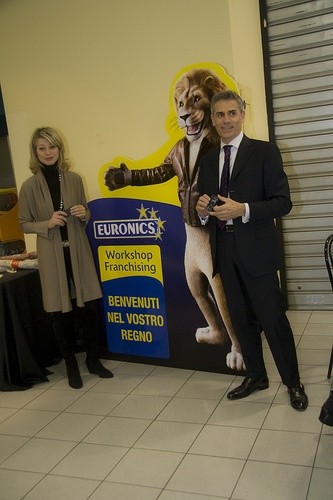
[0,254,62,392]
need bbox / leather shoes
[287,382,308,412]
[225,375,269,400]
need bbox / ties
[218,145,233,231]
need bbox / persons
[18,126,113,389]
[192,92,309,411]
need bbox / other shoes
[64,356,83,389]
[85,353,113,378]
